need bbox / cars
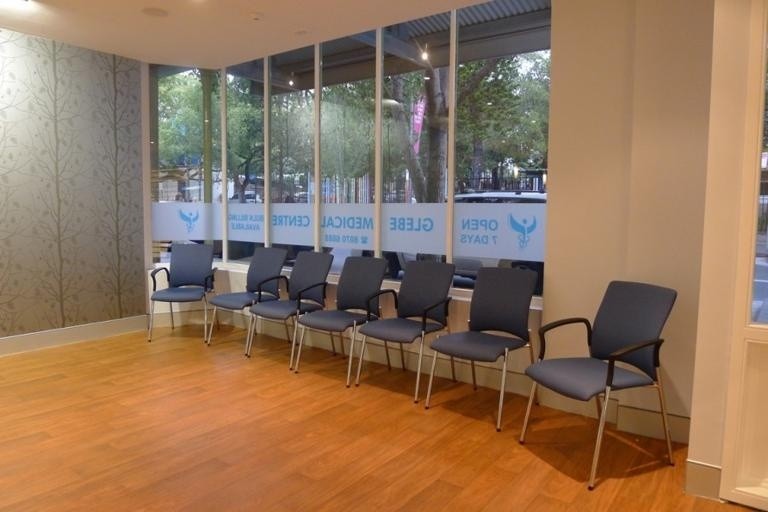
[229,191,262,203]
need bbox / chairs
[207,246,291,356]
[296,256,392,387]
[149,243,221,344]
[355,260,456,403]
[423,267,540,433]
[519,281,678,491]
[245,251,337,370]
